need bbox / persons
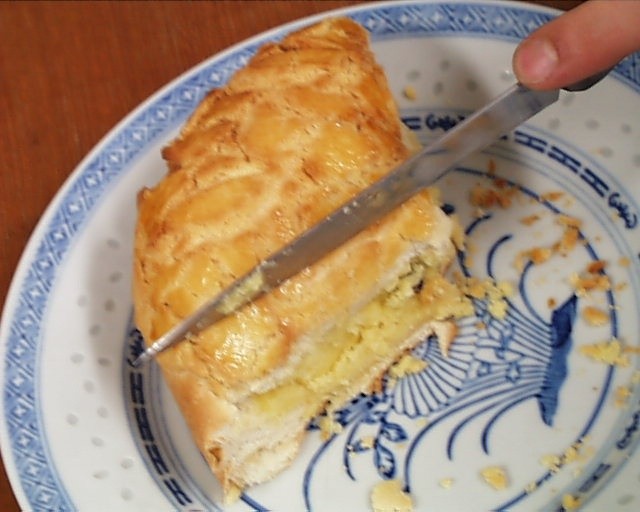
[511,1,640,94]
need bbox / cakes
[132,16,475,507]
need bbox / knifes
[131,64,617,367]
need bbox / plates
[0,1,639,512]
